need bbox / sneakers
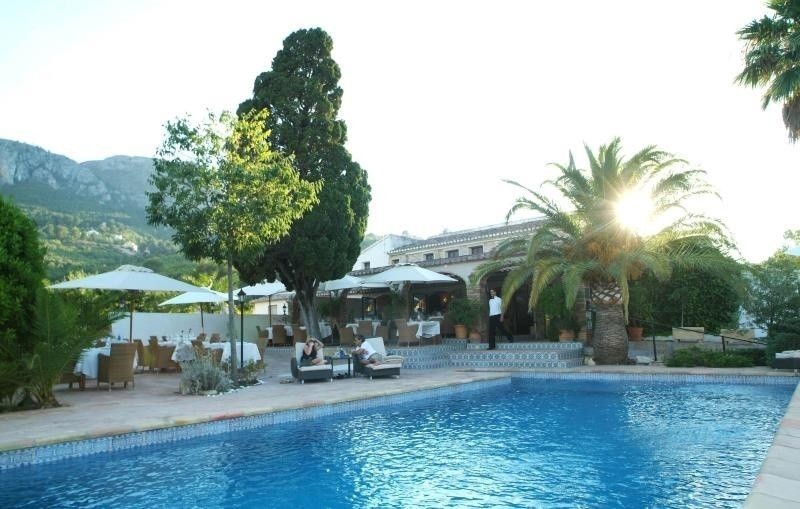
[509,337,513,343]
[487,347,495,350]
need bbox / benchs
[672,325,705,341]
[721,329,756,346]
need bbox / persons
[299,335,328,367]
[486,288,514,350]
[352,335,385,366]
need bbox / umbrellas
[45,264,217,345]
[232,277,296,328]
[316,262,459,346]
[159,287,238,333]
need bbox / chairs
[290,341,332,385]
[354,337,404,380]
[253,315,441,348]
[135,330,268,369]
[96,341,138,391]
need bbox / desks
[85,345,138,377]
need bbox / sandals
[279,376,291,383]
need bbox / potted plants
[447,294,484,339]
[538,284,579,341]
[625,282,649,338]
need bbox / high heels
[332,372,352,379]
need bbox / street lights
[238,287,246,368]
[283,303,286,325]
